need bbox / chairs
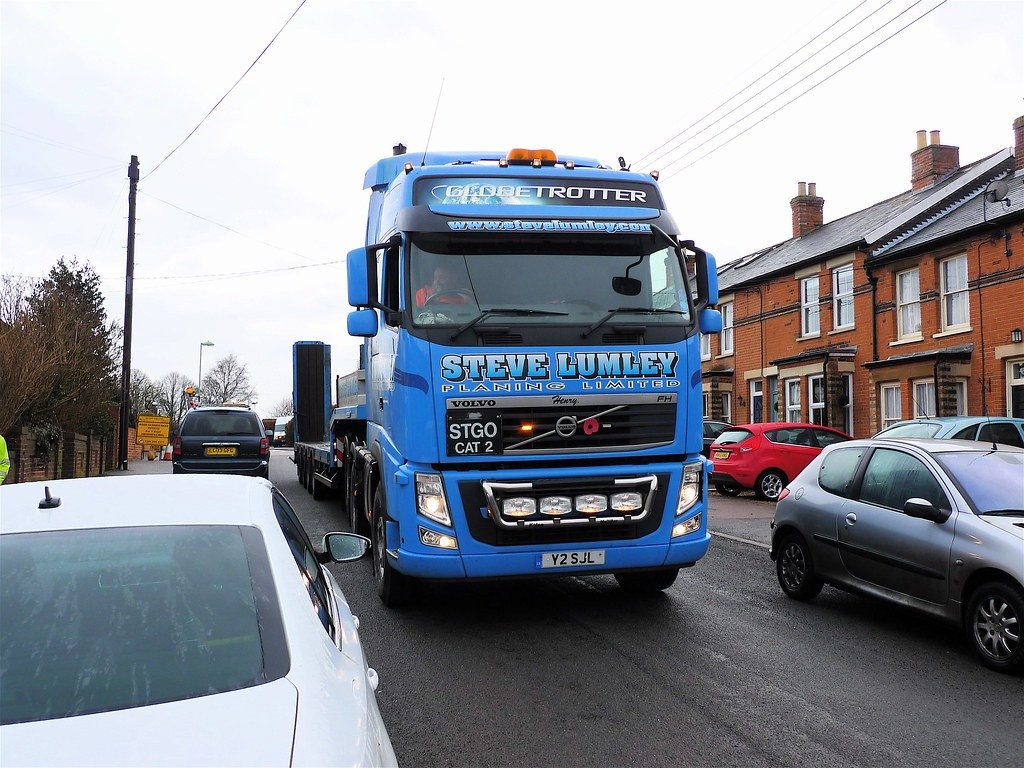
[196,418,213,436]
[777,432,789,442]
[796,432,809,445]
[233,419,250,434]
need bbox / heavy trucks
[288,148,724,610]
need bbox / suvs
[869,415,1024,460]
[172,404,274,480]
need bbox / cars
[764,440,1024,674]
[709,421,863,502]
[0,473,403,768]
[702,415,736,458]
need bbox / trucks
[273,416,294,447]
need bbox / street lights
[198,340,215,397]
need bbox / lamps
[1010,328,1022,343]
[737,395,745,407]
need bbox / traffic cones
[160,442,172,461]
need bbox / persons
[417,266,475,307]
[0,435,10,485]
